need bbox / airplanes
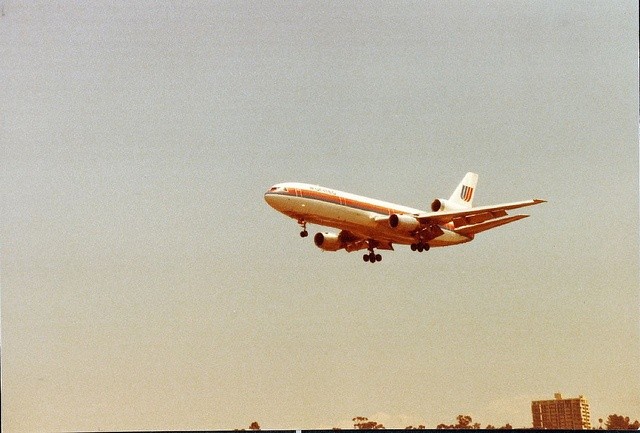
[265,171,549,263]
